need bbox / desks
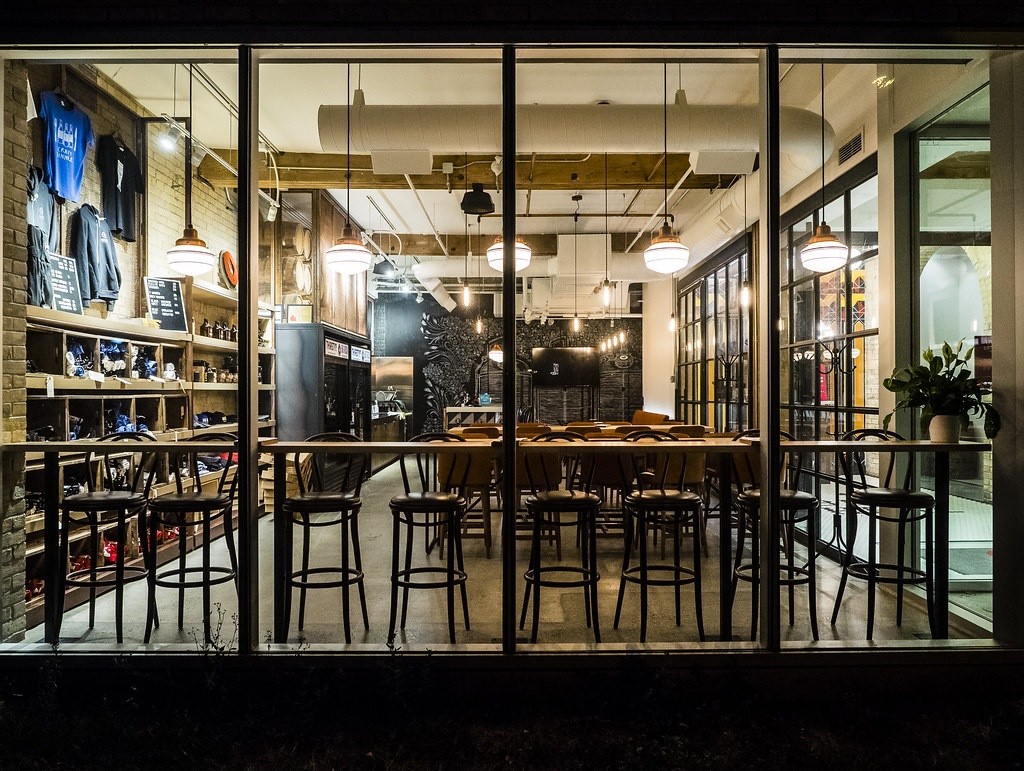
[6,438,278,640]
[258,436,991,646]
[449,422,716,434]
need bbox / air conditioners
[166,64,215,277]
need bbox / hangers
[111,124,127,148]
[51,68,75,106]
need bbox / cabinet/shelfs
[26,283,277,623]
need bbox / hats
[191,411,238,429]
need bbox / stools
[51,411,936,645]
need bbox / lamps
[487,228,532,272]
[643,62,690,275]
[800,63,848,272]
[323,63,372,275]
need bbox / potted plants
[882,336,1002,442]
